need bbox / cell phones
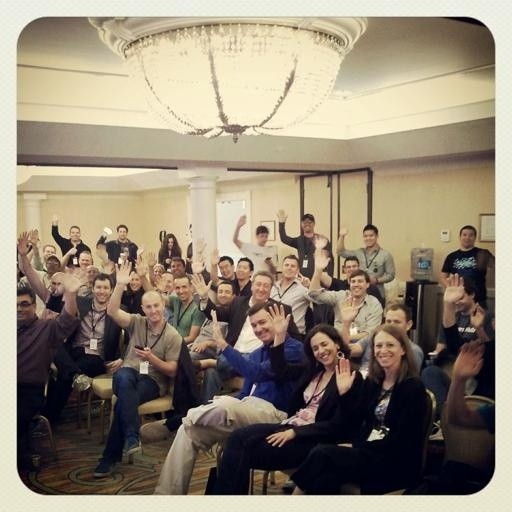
[135,345,144,351]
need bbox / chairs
[78,363,289,493]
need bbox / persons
[16,210,493,496]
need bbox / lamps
[88,20,369,141]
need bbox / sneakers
[191,358,213,371]
[93,458,112,477]
[72,373,92,392]
[127,439,141,456]
[139,419,174,440]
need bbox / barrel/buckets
[410,247,435,281]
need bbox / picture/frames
[480,214,496,243]
[260,220,276,241]
